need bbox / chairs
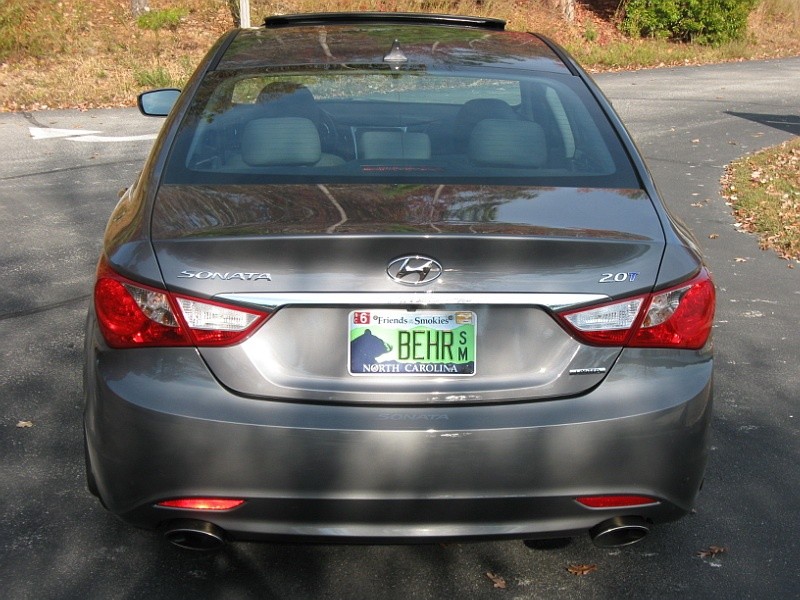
[215,119,348,173]
[254,78,323,127]
[443,95,522,132]
[467,117,549,171]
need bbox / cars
[84,13,715,575]
